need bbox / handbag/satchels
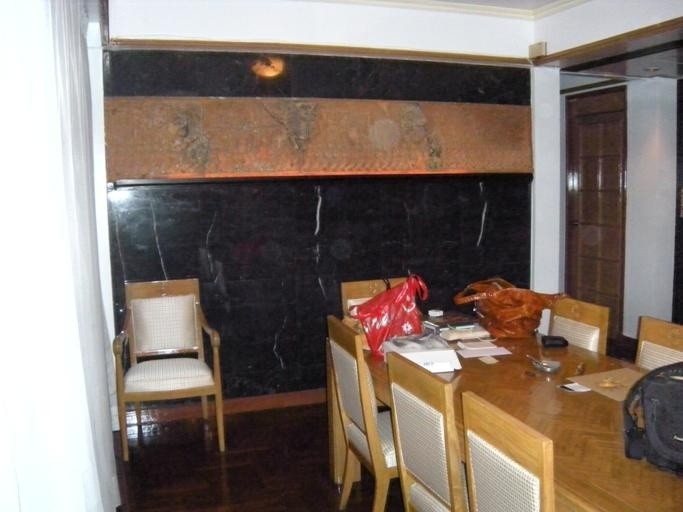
[454,279,570,339]
[623,363,682,473]
[347,276,429,359]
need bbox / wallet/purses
[542,336,568,347]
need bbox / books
[381,323,497,376]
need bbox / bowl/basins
[533,360,559,372]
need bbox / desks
[346,313,681,510]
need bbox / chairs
[340,277,409,322]
[635,315,682,375]
[327,314,405,511]
[532,292,551,337]
[462,389,556,512]
[549,294,609,357]
[382,348,470,511]
[113,277,225,461]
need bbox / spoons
[526,354,552,368]
